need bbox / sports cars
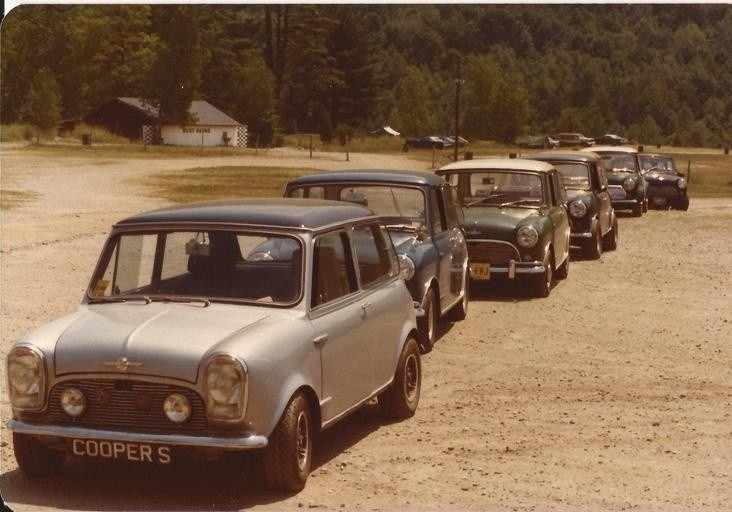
[519,136,560,149]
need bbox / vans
[558,133,595,146]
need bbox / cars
[5,198,421,490]
[494,153,618,260]
[246,169,469,351]
[576,147,648,218]
[421,159,570,297]
[610,154,688,211]
[405,137,469,149]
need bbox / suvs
[603,134,628,145]
